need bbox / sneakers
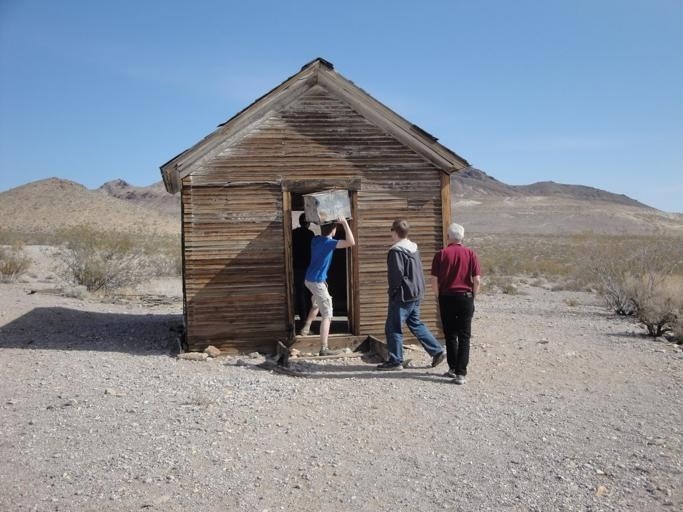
[377,362,403,371]
[448,369,467,384]
[432,351,447,367]
[319,347,338,356]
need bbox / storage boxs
[301,186,353,226]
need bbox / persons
[298,214,356,356]
[431,222,481,385]
[375,219,446,371]
[292,212,315,321]
